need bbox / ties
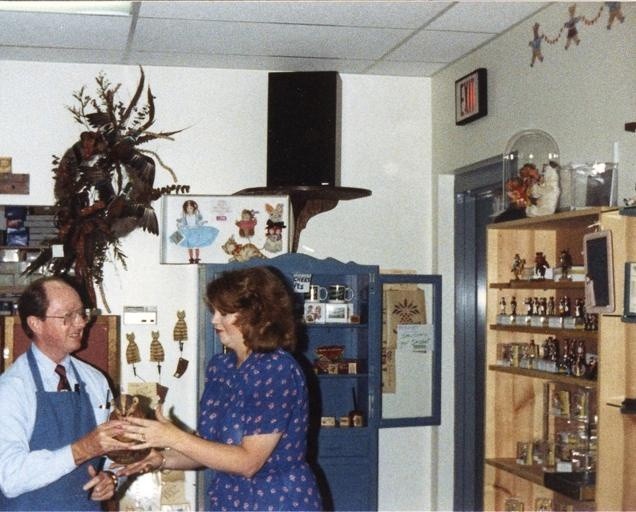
[55,366,71,393]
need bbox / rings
[141,434,147,442]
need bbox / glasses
[41,308,92,327]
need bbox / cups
[304,283,355,304]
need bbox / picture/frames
[4,315,119,406]
[379,274,441,429]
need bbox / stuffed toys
[524,160,562,219]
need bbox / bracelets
[101,470,118,488]
[157,452,167,473]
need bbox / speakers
[269,72,341,187]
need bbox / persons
[166,199,219,265]
[0,276,140,512]
[116,265,326,512]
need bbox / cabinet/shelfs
[198,253,381,510]
[482,205,636,512]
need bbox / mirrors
[584,224,616,316]
